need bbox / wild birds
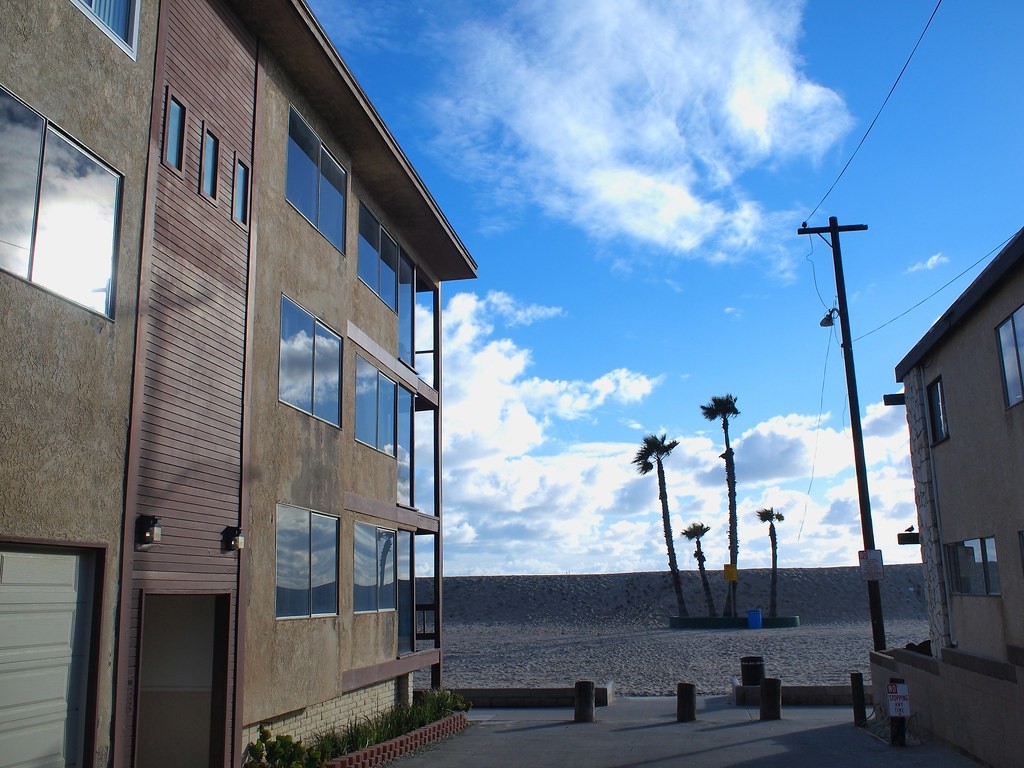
[905,525,914,532]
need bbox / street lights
[820,307,886,653]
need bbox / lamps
[224,527,246,551]
[135,514,163,545]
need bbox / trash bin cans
[746,609,762,629]
[740,655,766,686]
